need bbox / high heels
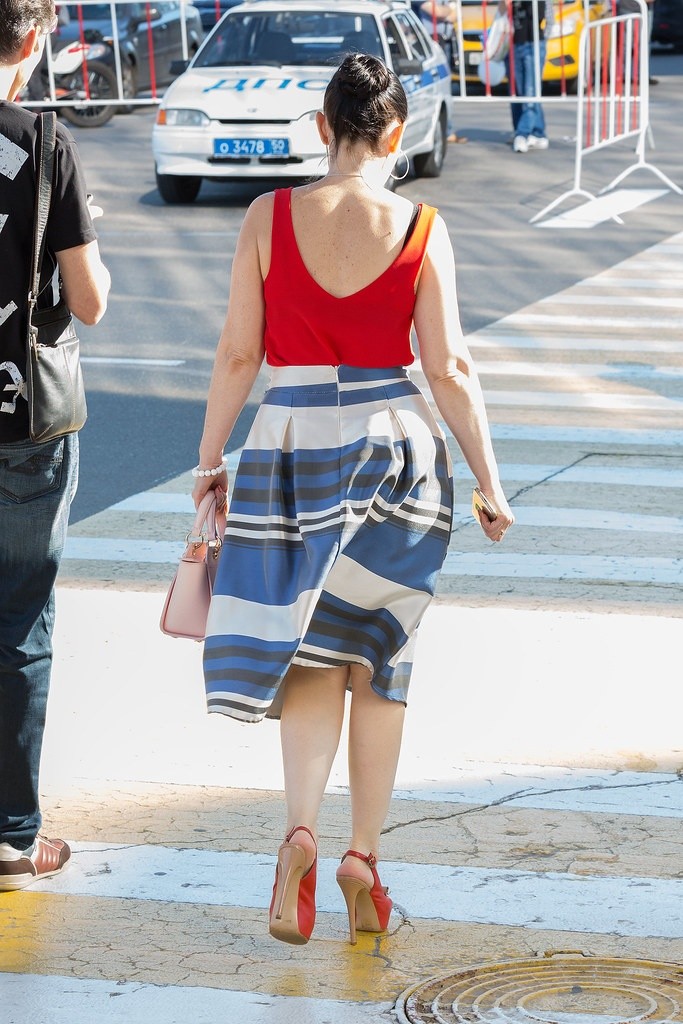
[269,826,317,945]
[336,849,393,946]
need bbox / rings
[498,528,505,538]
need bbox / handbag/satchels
[26,111,88,445]
[485,2,515,62]
[160,489,228,643]
[479,27,506,87]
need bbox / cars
[149,0,454,206]
[41,0,207,113]
[448,0,609,84]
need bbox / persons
[0,0,110,891]
[411,1,467,144]
[499,1,554,153]
[192,51,514,949]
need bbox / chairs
[341,29,380,57]
[255,33,295,62]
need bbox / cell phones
[471,487,497,525]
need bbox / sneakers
[513,135,529,153]
[0,832,72,891]
[527,134,549,150]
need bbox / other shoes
[637,77,658,86]
[447,133,469,145]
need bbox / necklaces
[324,175,369,179]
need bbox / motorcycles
[13,27,121,128]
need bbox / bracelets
[192,456,227,477]
